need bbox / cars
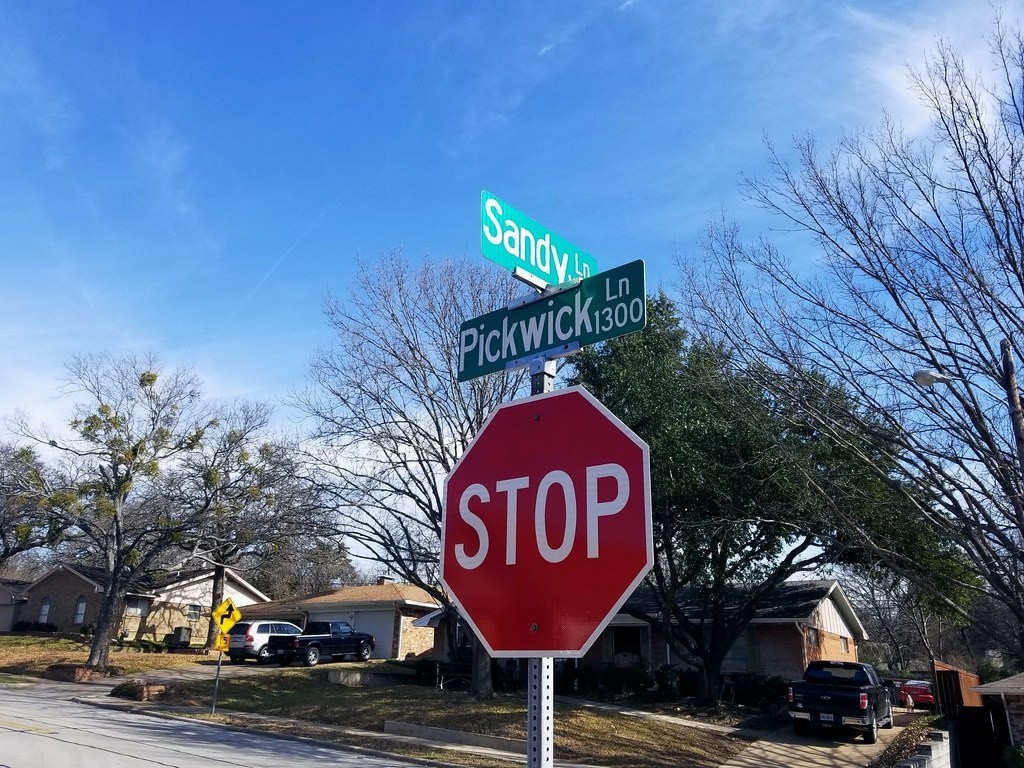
[899,680,935,709]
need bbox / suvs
[222,619,305,664]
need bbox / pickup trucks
[788,659,894,745]
[266,620,377,667]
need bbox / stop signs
[439,385,657,660]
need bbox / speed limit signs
[215,633,231,652]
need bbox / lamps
[59,566,63,571]
[93,587,98,593]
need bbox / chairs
[852,672,865,681]
[821,671,832,677]
